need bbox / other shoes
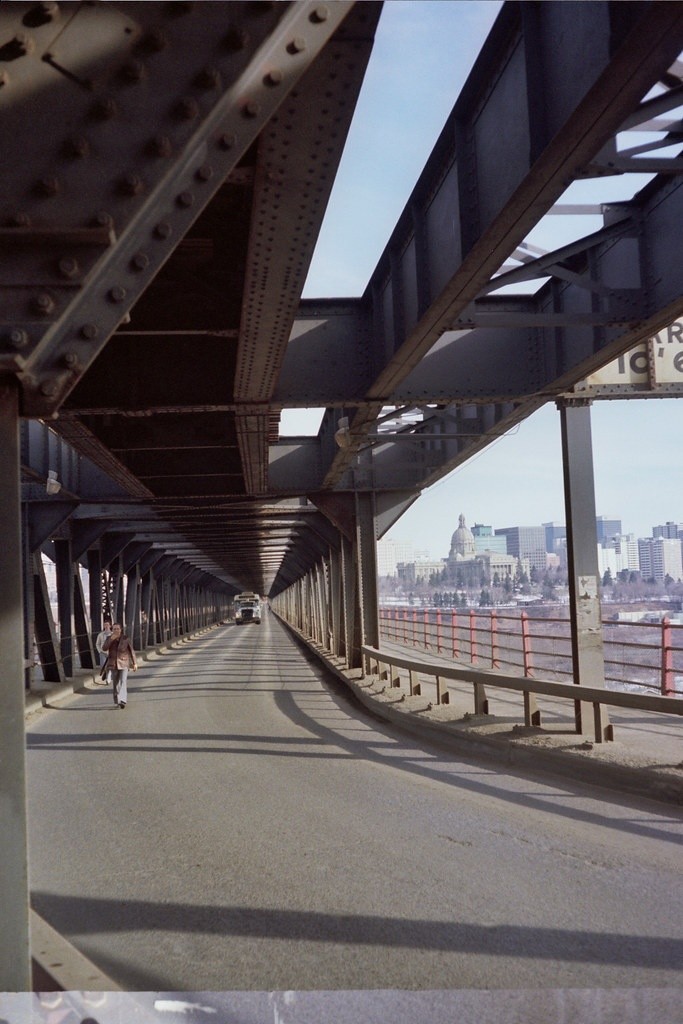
[119,702,125,709]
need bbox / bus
[234,592,262,625]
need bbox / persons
[268,604,271,616]
[103,623,138,709]
[96,620,112,685]
